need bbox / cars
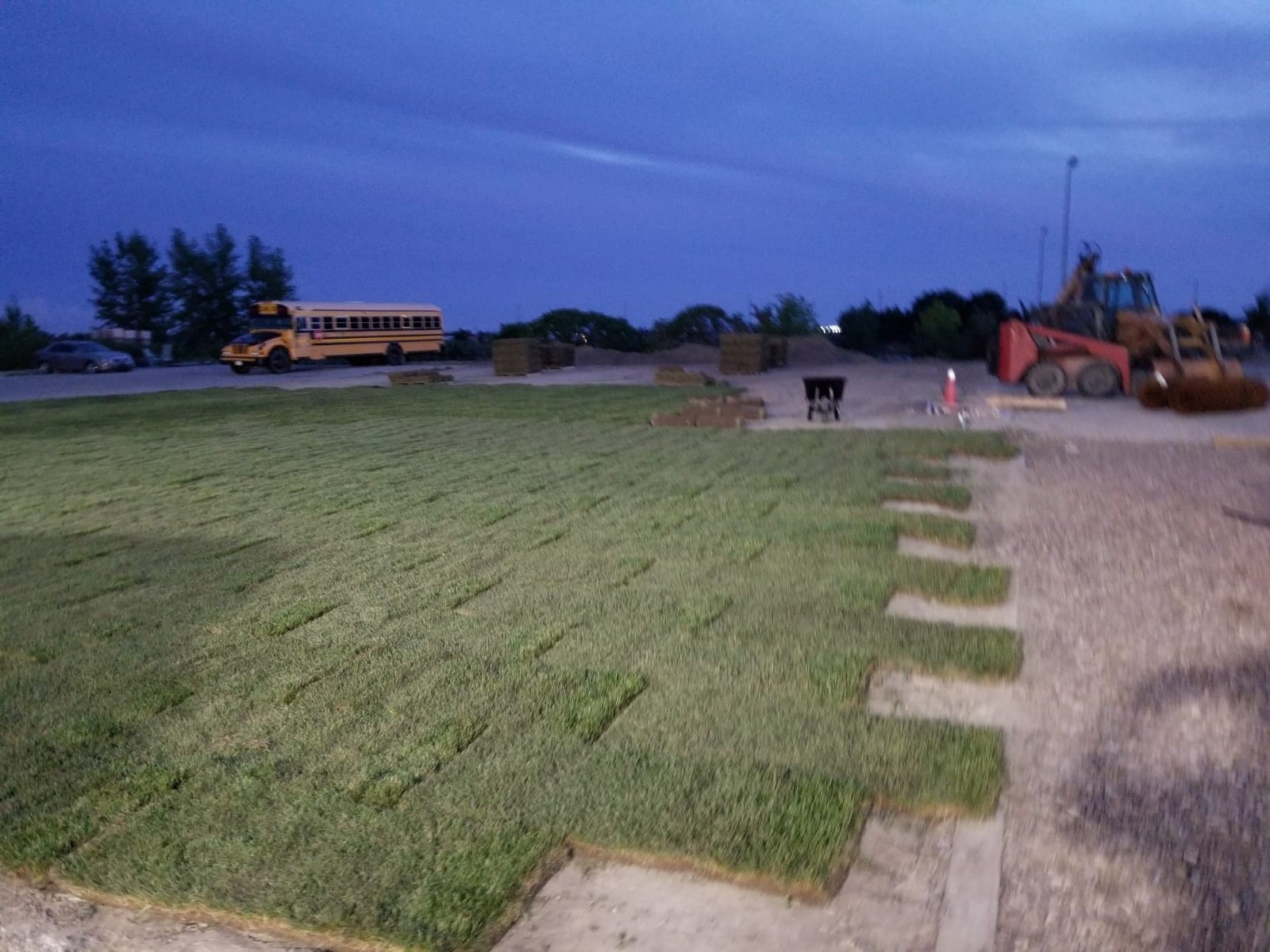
[25,340,137,375]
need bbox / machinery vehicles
[984,259,1270,416]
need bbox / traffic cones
[937,367,962,410]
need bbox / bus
[219,299,446,373]
